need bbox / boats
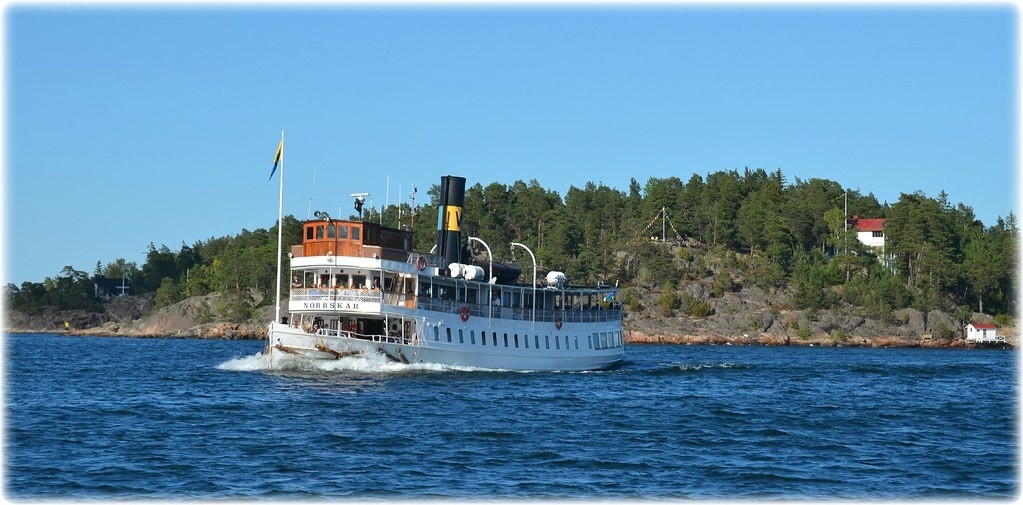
[467,254,523,284]
[262,128,628,374]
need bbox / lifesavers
[388,320,402,333]
[555,315,563,329]
[416,255,428,272]
[461,305,470,321]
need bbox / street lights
[835,192,849,261]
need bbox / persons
[292,275,384,303]
[293,320,324,335]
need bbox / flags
[268,140,282,181]
